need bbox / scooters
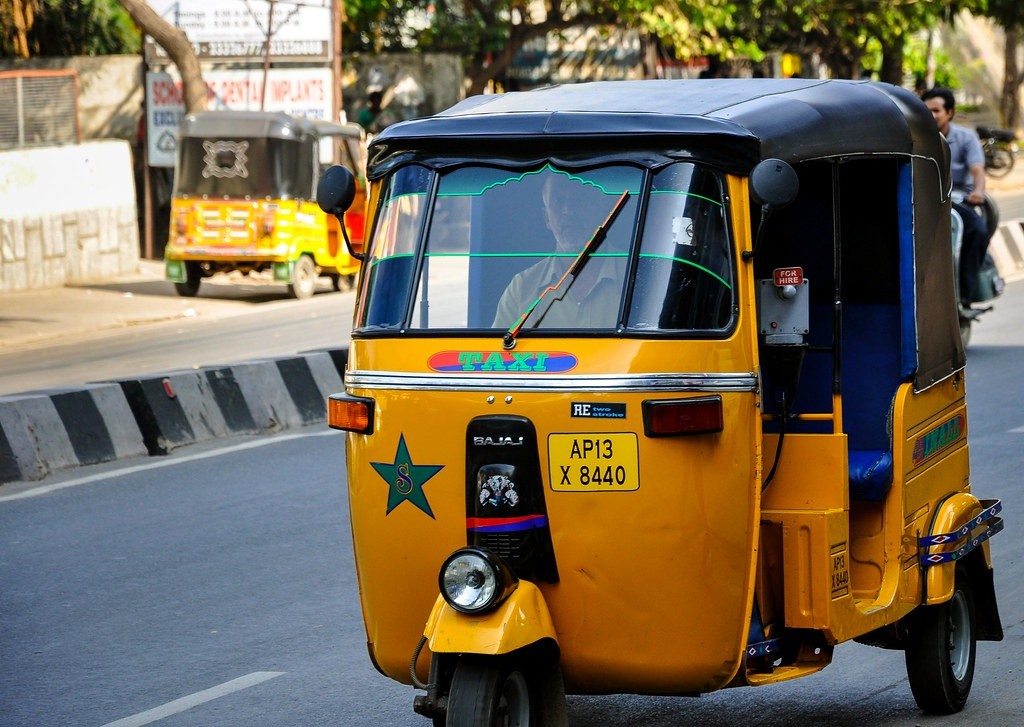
[950,188,1000,322]
[973,124,1017,179]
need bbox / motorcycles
[162,109,388,299]
[316,72,1004,727]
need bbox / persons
[490,174,628,328]
[920,88,988,311]
